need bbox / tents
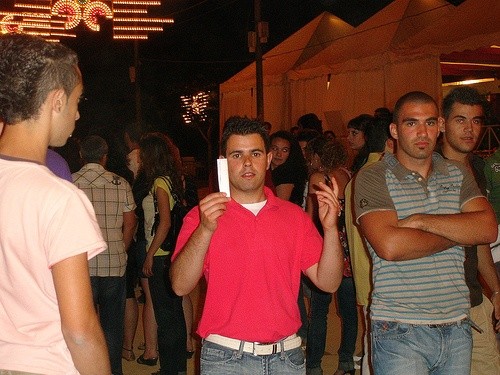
[220,1,499,166]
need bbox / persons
[45,85,500,375]
[0,33,112,375]
[354,92,500,375]
[169,117,344,374]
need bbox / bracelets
[490,292,500,300]
